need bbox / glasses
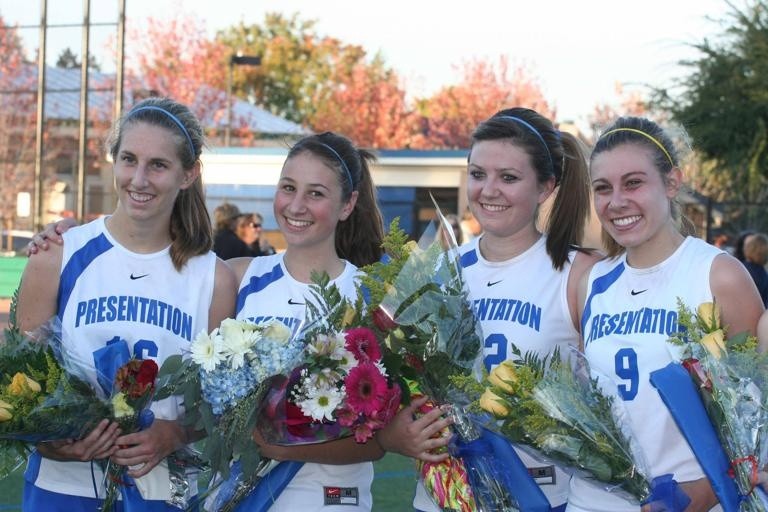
[247,222,262,228]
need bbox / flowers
[0,361,46,426]
[673,291,768,421]
[286,233,438,451]
[179,307,294,379]
[470,354,557,443]
[92,349,171,434]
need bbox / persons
[563,116,767,511]
[434,214,463,249]
[715,232,768,307]
[376,107,605,512]
[211,203,277,261]
[25,130,386,512]
[16,97,239,511]
